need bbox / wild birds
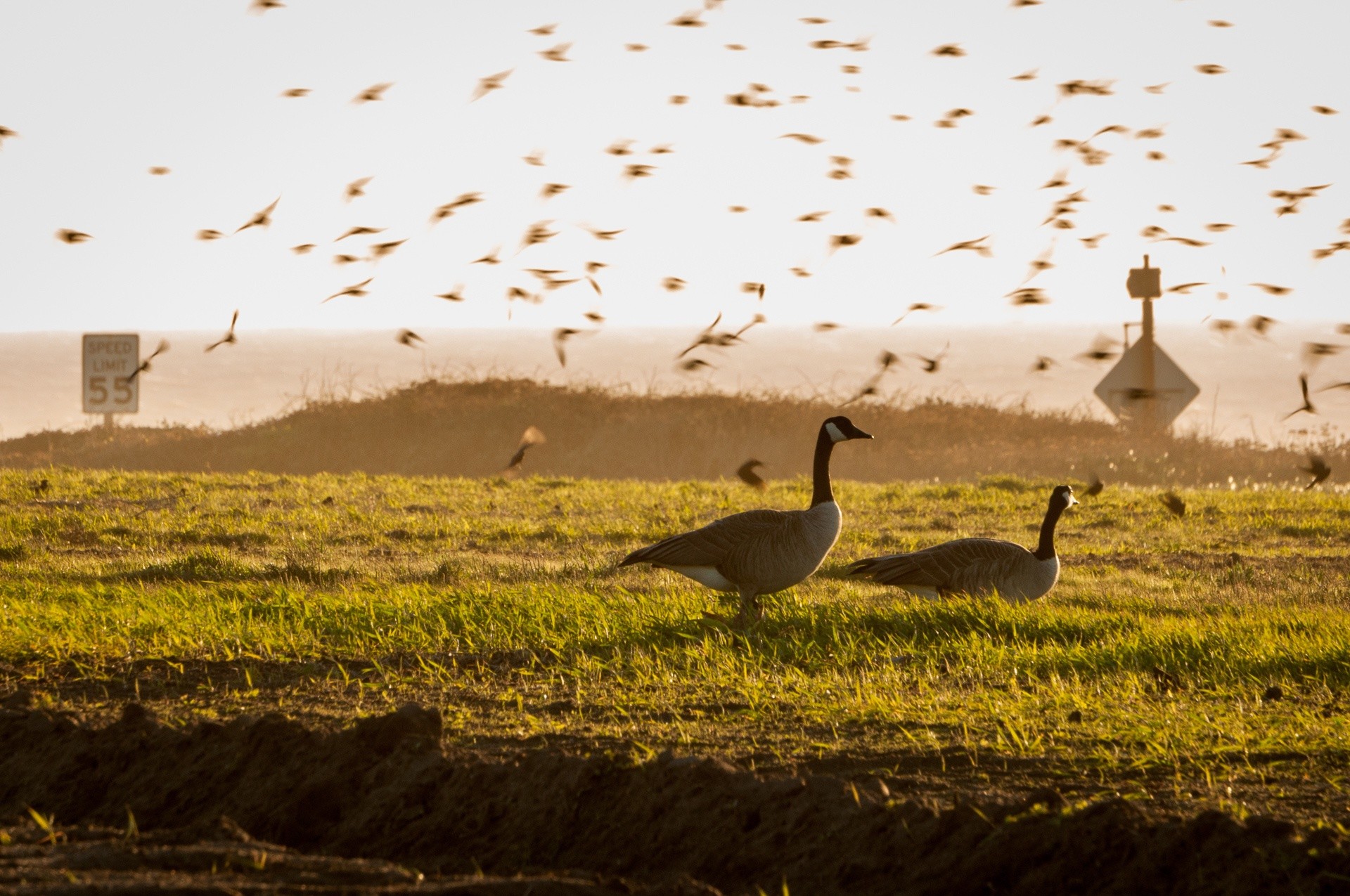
[2,0,1350,519]
[843,485,1080,605]
[615,416,876,617]
